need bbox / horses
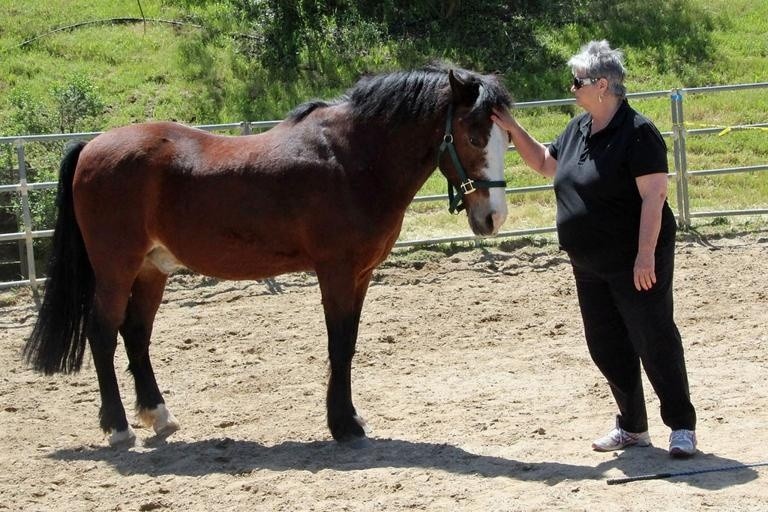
[21,60,512,449]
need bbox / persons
[488,39,697,457]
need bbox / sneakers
[668,429,697,456]
[591,414,650,451]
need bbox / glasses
[573,77,598,91]
